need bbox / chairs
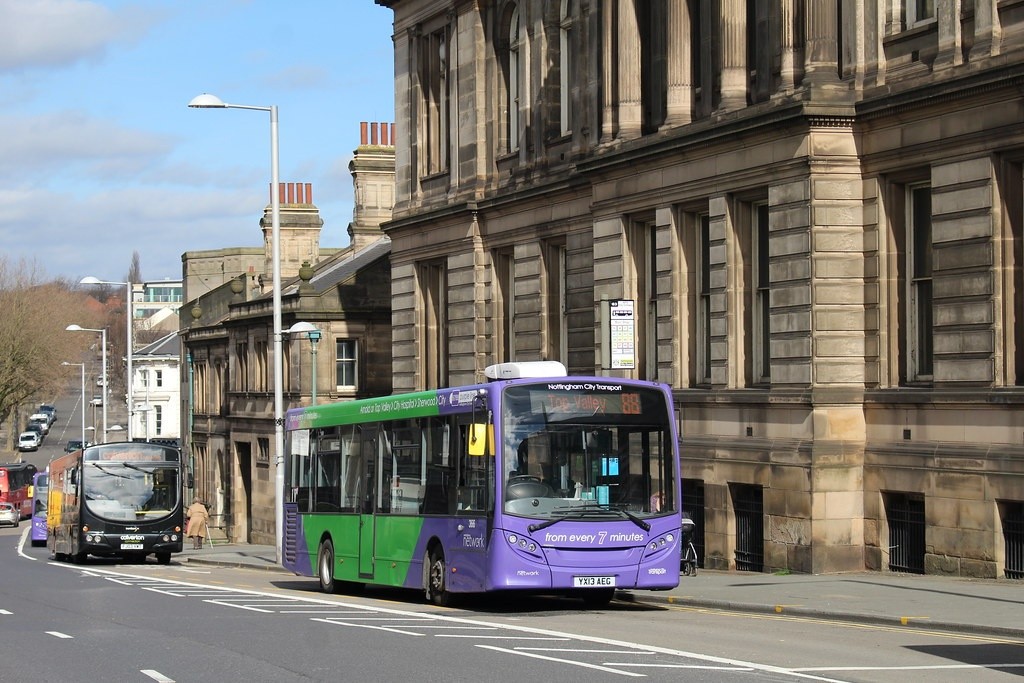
[298,467,487,513]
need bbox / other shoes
[195,547,198,550]
[199,546,202,549]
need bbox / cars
[96,375,109,387]
[15,404,58,452]
[0,501,21,527]
[92,394,102,407]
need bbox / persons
[187,497,209,549]
[650,490,665,512]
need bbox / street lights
[186,92,320,566]
[60,361,96,449]
[64,324,124,444]
[78,276,153,441]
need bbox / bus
[280,360,685,607]
[0,460,41,521]
[30,471,50,547]
[46,438,195,565]
